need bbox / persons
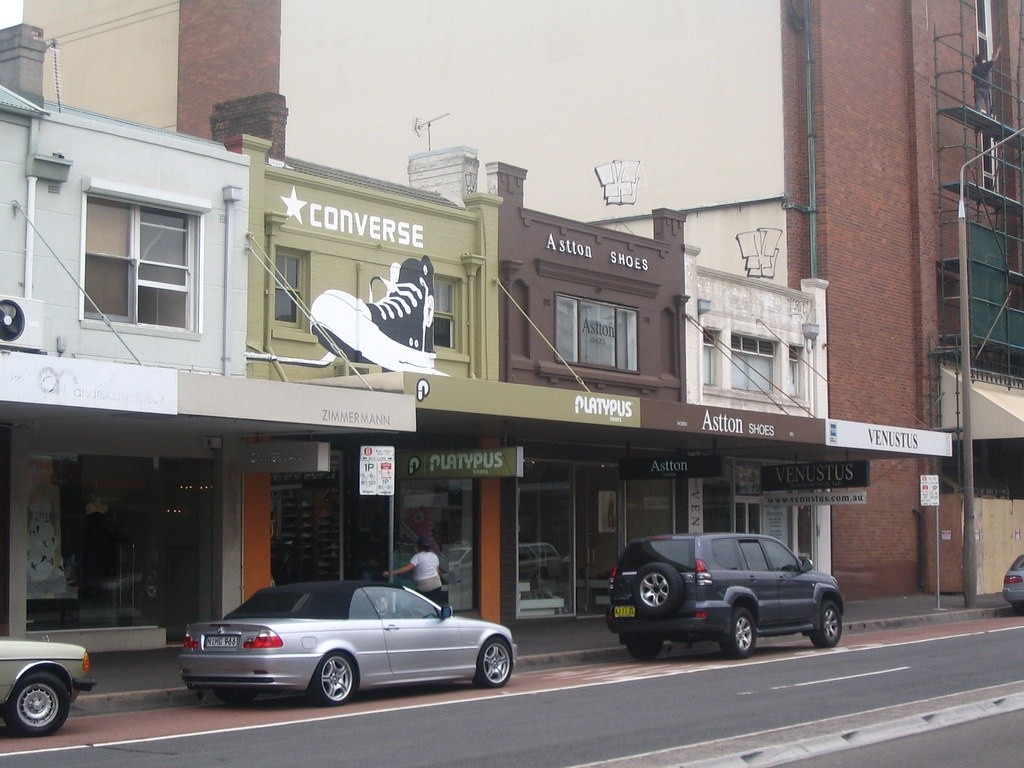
[86,495,115,580]
[971,46,1001,117]
[608,493,614,530]
[383,537,442,602]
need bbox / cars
[0,635,97,738]
[175,578,518,707]
[517,541,570,588]
[448,547,473,582]
[1002,554,1024,616]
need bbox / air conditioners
[0,295,44,351]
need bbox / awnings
[939,364,1024,441]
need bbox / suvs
[604,530,846,662]
